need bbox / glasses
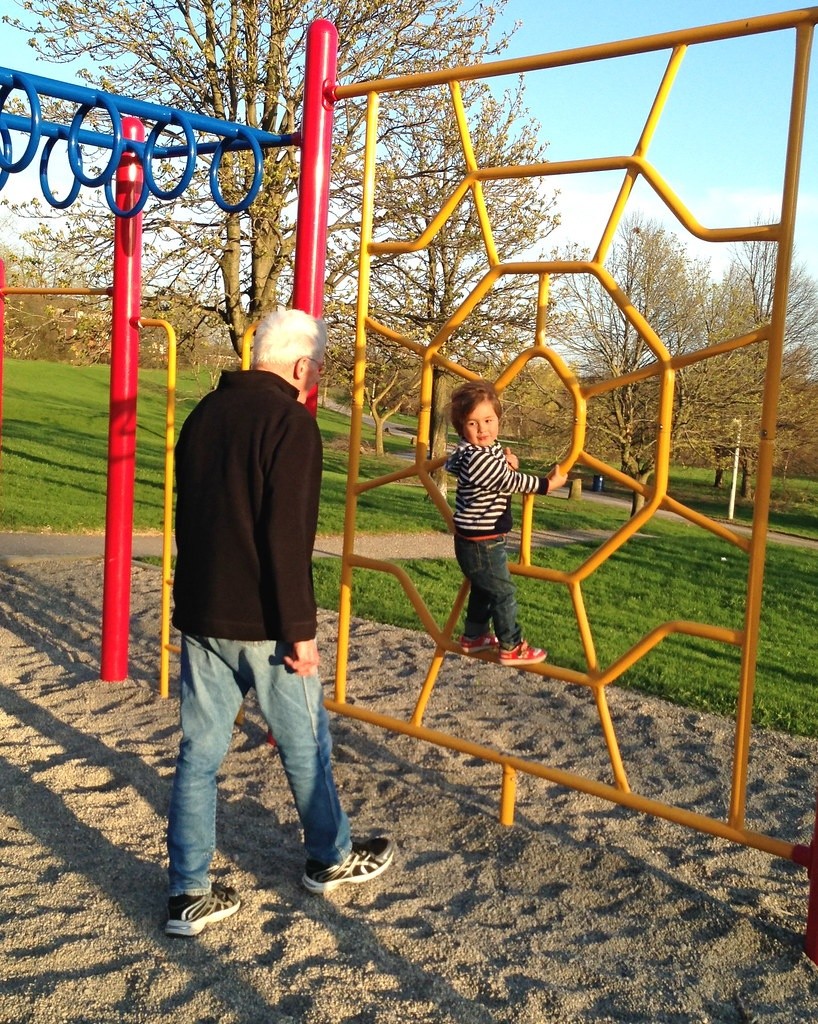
[308,356,327,374]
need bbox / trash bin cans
[593,474,605,492]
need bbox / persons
[164,310,395,937]
[444,380,567,666]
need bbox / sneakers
[301,836,396,893]
[498,639,548,665]
[459,632,500,653]
[165,881,241,936]
